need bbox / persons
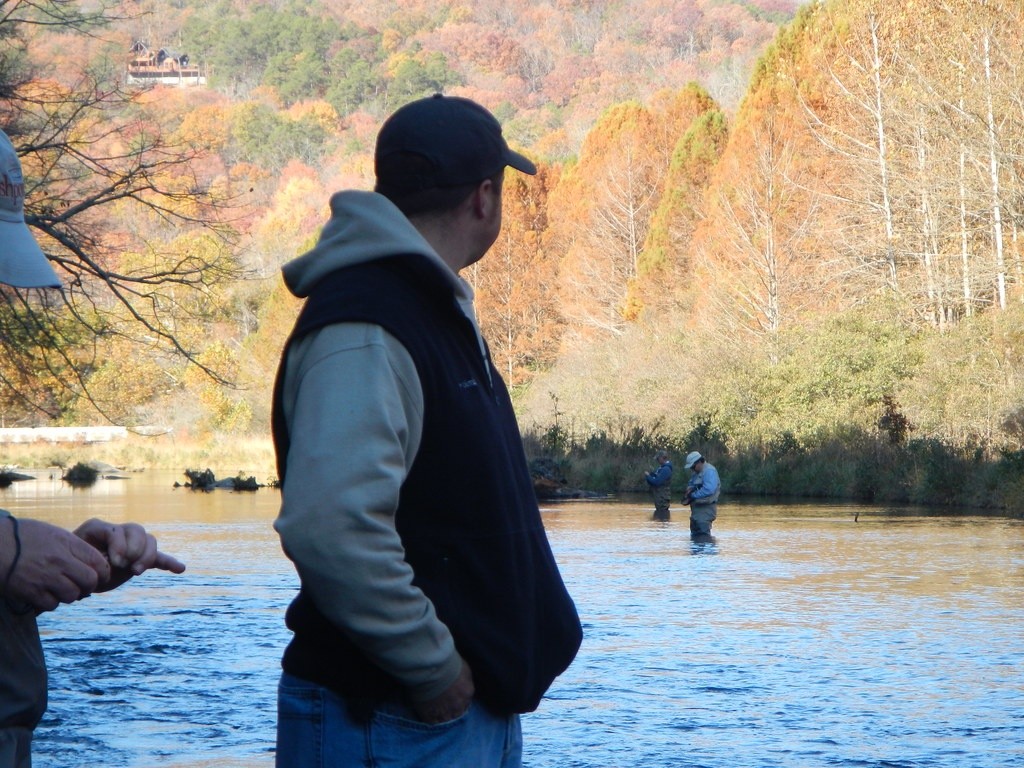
[681,451,721,543]
[270,93,583,768]
[0,132,184,768]
[644,450,672,511]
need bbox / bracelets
[0,515,22,593]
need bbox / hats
[0,128,62,289]
[652,450,668,460]
[374,93,537,186]
[684,451,701,469]
